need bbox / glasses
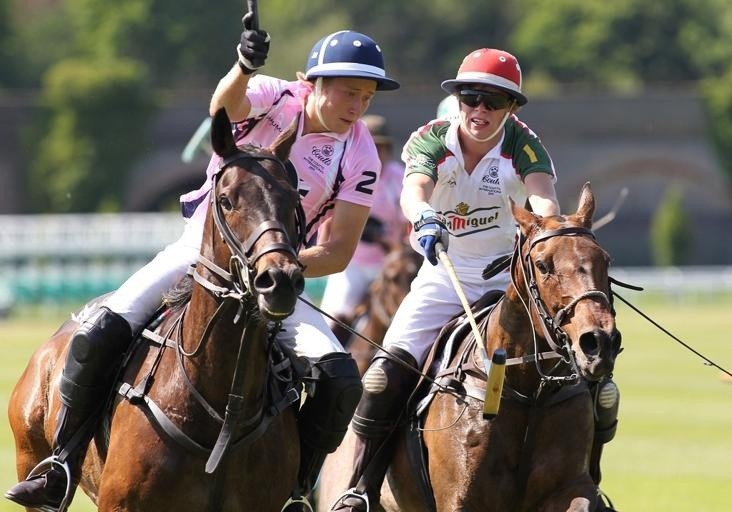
[459,89,507,111]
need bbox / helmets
[440,48,528,107]
[305,29,400,91]
[359,114,395,147]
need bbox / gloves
[409,210,449,266]
[237,1,271,70]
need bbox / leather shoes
[596,493,615,511]
[333,488,380,511]
[4,469,82,512]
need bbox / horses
[328,225,424,378]
[7,103,305,512]
[379,181,625,512]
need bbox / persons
[1,0,400,512]
[319,113,411,346]
[328,45,618,511]
